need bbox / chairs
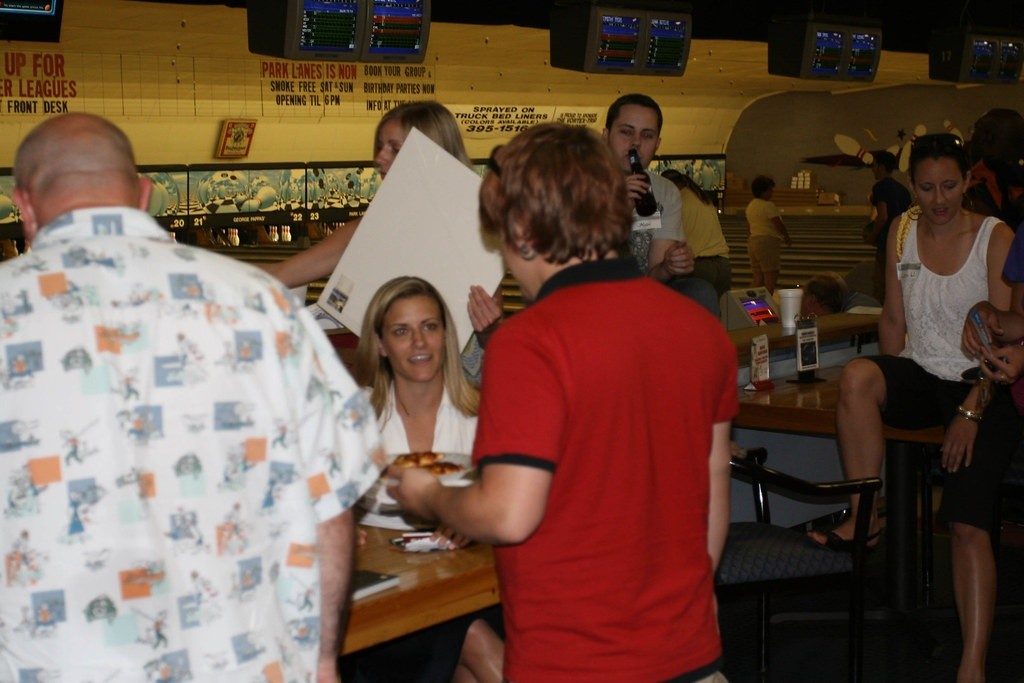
[715,444,885,683]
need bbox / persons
[263,97,508,392]
[336,276,505,681]
[0,112,386,681]
[595,93,722,329]
[655,109,1024,683]
[379,121,739,681]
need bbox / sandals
[805,528,882,562]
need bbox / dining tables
[340,523,501,663]
[719,363,1024,662]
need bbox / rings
[998,372,1003,383]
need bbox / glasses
[912,133,963,153]
[487,145,503,177]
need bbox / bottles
[627,148,657,217]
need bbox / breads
[392,451,465,477]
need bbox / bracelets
[957,406,984,424]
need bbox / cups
[778,288,804,328]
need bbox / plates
[373,451,475,487]
